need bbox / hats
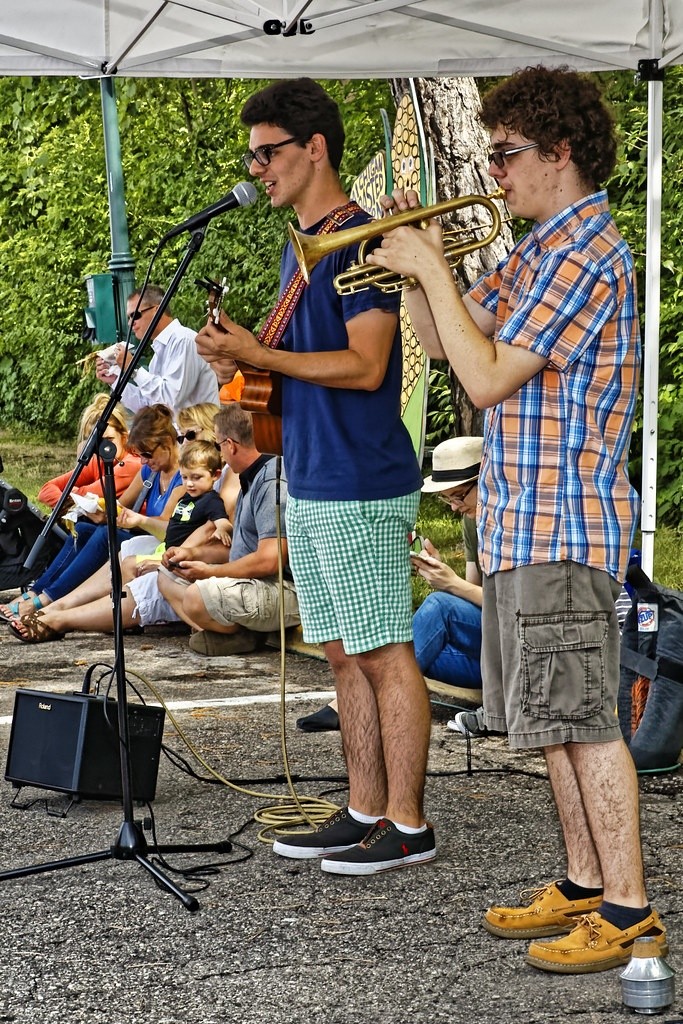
[416,436,483,493]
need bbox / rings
[140,567,144,570]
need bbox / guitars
[190,273,283,458]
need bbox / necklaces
[164,488,166,493]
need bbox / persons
[0,393,301,655]
[195,77,435,874]
[95,286,219,436]
[295,436,484,732]
[365,63,668,973]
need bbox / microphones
[158,182,259,248]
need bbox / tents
[0,0,683,582]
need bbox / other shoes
[297,706,340,731]
[188,629,256,655]
[455,706,498,736]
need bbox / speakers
[5,690,165,805]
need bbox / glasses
[439,482,477,506]
[177,429,203,444]
[489,144,539,168]
[128,306,155,320]
[214,438,239,450]
[243,138,294,167]
[134,442,160,458]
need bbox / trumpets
[286,186,509,297]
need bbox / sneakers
[524,909,671,973]
[271,807,376,861]
[485,880,605,939]
[320,819,437,876]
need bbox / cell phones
[409,551,432,567]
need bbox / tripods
[0,223,232,913]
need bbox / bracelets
[130,365,140,378]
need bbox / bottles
[620,936,676,1014]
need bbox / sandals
[7,616,65,643]
[14,611,45,621]
[0,597,42,620]
[9,593,32,604]
